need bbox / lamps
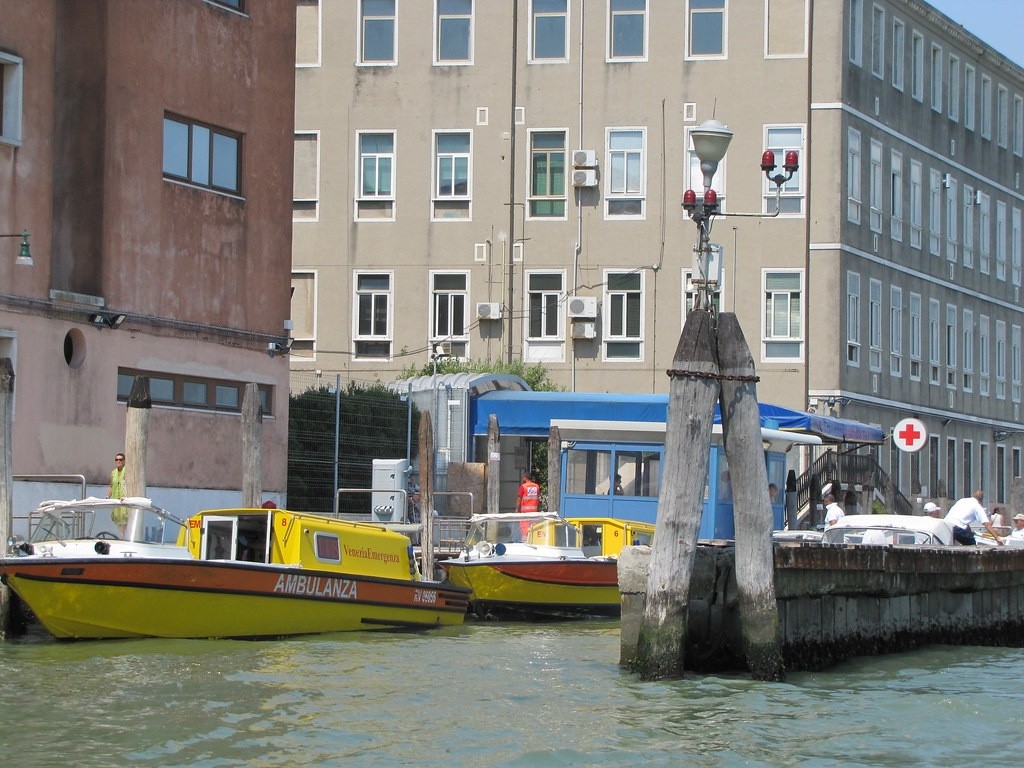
[88,313,127,330]
[941,419,951,426]
[269,338,294,358]
[823,396,853,410]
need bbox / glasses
[115,459,123,461]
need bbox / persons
[722,471,733,499]
[607,474,624,495]
[944,490,1005,545]
[1012,513,1024,538]
[769,484,779,503]
[408,477,438,523]
[104,453,128,539]
[989,507,1004,534]
[923,502,941,518]
[516,472,540,535]
[823,494,845,543]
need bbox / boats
[0,494,473,640]
[435,510,657,621]
[696,513,955,547]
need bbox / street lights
[688,91,733,307]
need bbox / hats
[924,502,941,513]
[1012,514,1024,521]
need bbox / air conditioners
[572,150,598,167]
[476,303,502,320]
[572,322,597,338]
[568,296,598,318]
[572,170,598,187]
